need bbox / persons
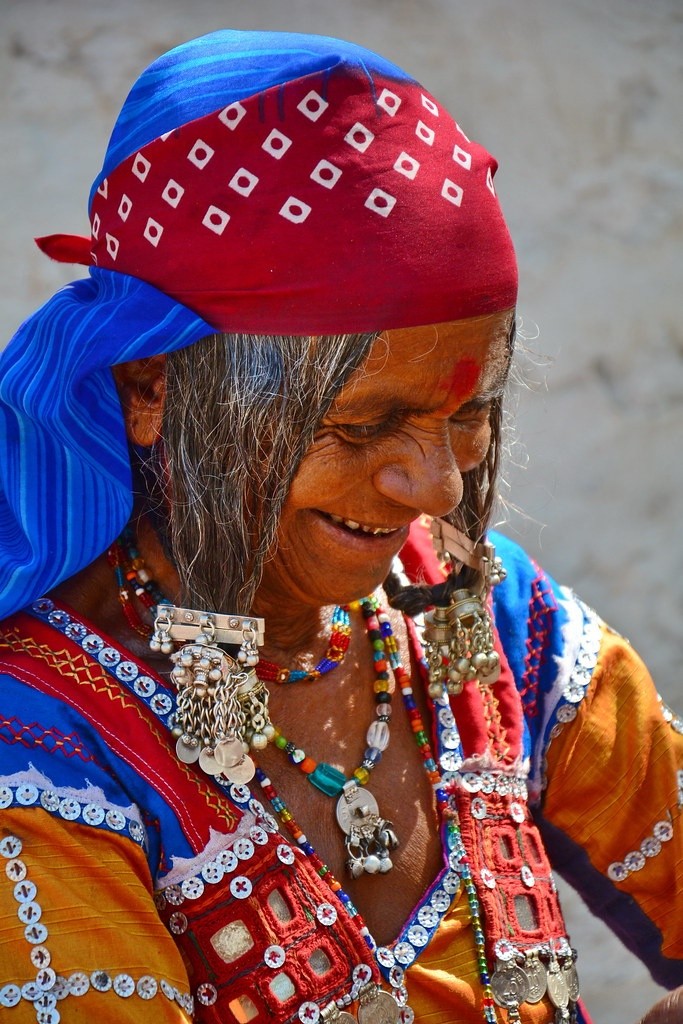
[0,32,683,1024]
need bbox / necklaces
[104,530,497,1024]
